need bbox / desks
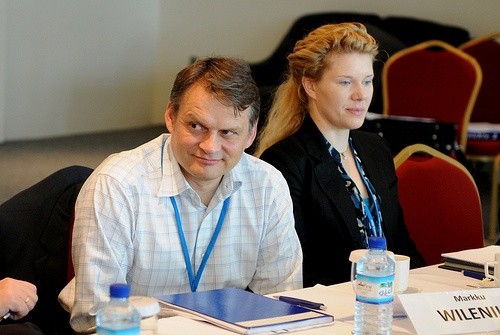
[141,262,500,335]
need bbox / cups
[349,249,410,305]
[129,295,162,335]
[484,253,500,286]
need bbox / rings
[24,297,30,303]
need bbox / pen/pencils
[279,296,327,311]
[461,270,485,280]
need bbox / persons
[256,23,424,288]
[0,278,38,320]
[57,56,305,332]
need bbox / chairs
[381,33,500,266]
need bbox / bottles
[95,283,140,334]
[352,237,395,335]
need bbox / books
[154,288,334,335]
[438,257,494,275]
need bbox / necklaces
[341,146,350,159]
